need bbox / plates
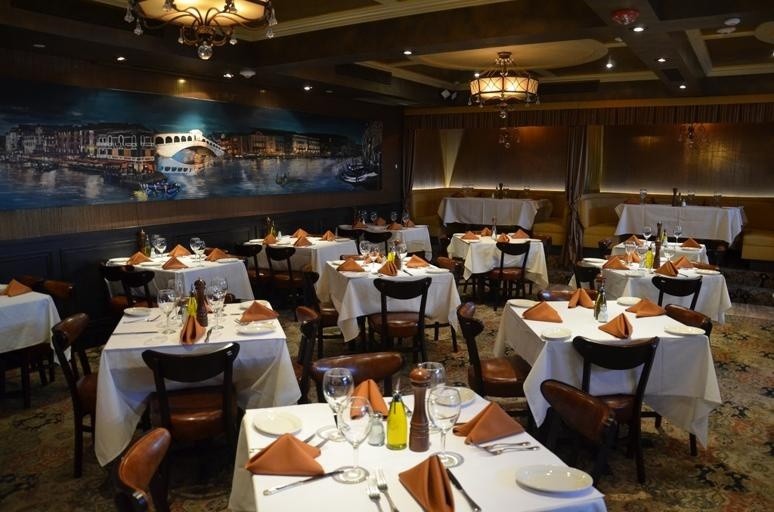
[696,270,720,275]
[617,296,641,306]
[335,239,351,242]
[541,327,572,340]
[582,257,605,263]
[216,259,238,263]
[236,321,275,334]
[665,324,705,336]
[248,239,264,243]
[276,242,290,245]
[140,262,161,267]
[513,463,593,493]
[253,410,303,435]
[123,307,152,317]
[509,298,536,308]
[626,270,645,277]
[109,259,130,262]
[427,268,450,274]
[682,246,699,251]
[436,386,475,408]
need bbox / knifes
[263,470,344,497]
[447,469,481,512]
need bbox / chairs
[367,277,433,362]
[303,269,367,359]
[336,226,363,260]
[652,275,703,311]
[488,240,531,312]
[119,267,155,308]
[542,235,552,259]
[641,304,713,457]
[141,342,240,471]
[310,352,406,404]
[532,380,618,489]
[599,240,614,260]
[118,427,172,512]
[279,307,323,401]
[573,336,660,483]
[233,241,271,298]
[16,275,49,386]
[100,262,135,308]
[457,301,534,438]
[363,230,392,256]
[572,264,600,291]
[52,312,151,464]
[434,257,464,289]
[38,279,92,386]
[264,246,320,321]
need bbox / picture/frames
[0,81,385,212]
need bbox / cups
[359,241,371,268]
[337,397,374,483]
[190,238,201,262]
[360,210,367,220]
[643,225,653,245]
[369,245,380,271]
[156,237,167,263]
[195,241,206,267]
[168,279,183,301]
[637,245,647,269]
[625,241,637,267]
[157,289,176,335]
[211,277,228,317]
[640,188,647,205]
[397,244,407,267]
[664,246,675,261]
[391,212,397,223]
[371,213,376,221]
[152,235,160,258]
[419,362,446,435]
[426,387,461,469]
[673,225,682,245]
[322,368,354,441]
[402,212,409,229]
[206,286,224,330]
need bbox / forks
[483,446,539,455]
[366,486,383,512]
[472,442,531,450]
[376,472,399,512]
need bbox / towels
[511,228,529,238]
[374,217,386,226]
[673,256,693,269]
[293,228,308,239]
[405,255,429,268]
[480,228,492,236]
[179,314,205,346]
[399,455,456,512]
[204,248,228,261]
[598,312,633,339]
[625,298,667,317]
[127,252,151,266]
[602,255,630,270]
[403,219,414,227]
[656,261,678,277]
[352,223,368,228]
[497,233,510,242]
[388,224,401,230]
[167,244,192,257]
[461,230,479,240]
[625,235,640,245]
[680,237,702,248]
[162,256,188,270]
[262,234,277,245]
[244,433,324,476]
[568,288,595,309]
[378,260,397,276]
[336,258,365,271]
[1,279,32,297]
[522,301,563,323]
[622,251,640,263]
[350,379,389,419]
[239,301,279,324]
[322,231,335,241]
[452,401,525,444]
[293,235,312,247]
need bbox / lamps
[494,126,521,152]
[124,0,278,60]
[467,52,539,118]
[679,123,711,152]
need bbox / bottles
[409,368,432,452]
[143,235,150,257]
[672,187,678,207]
[495,186,499,199]
[661,228,667,245]
[677,192,682,206]
[394,240,401,270]
[595,289,607,320]
[492,193,494,199]
[682,200,686,207]
[140,231,145,254]
[653,239,661,269]
[598,304,609,322]
[195,280,208,327]
[182,304,189,323]
[271,220,276,239]
[498,182,503,199]
[151,248,155,259]
[189,291,197,319]
[278,230,281,241]
[387,389,407,449]
[388,247,395,263]
[645,247,654,269]
[656,223,662,246]
